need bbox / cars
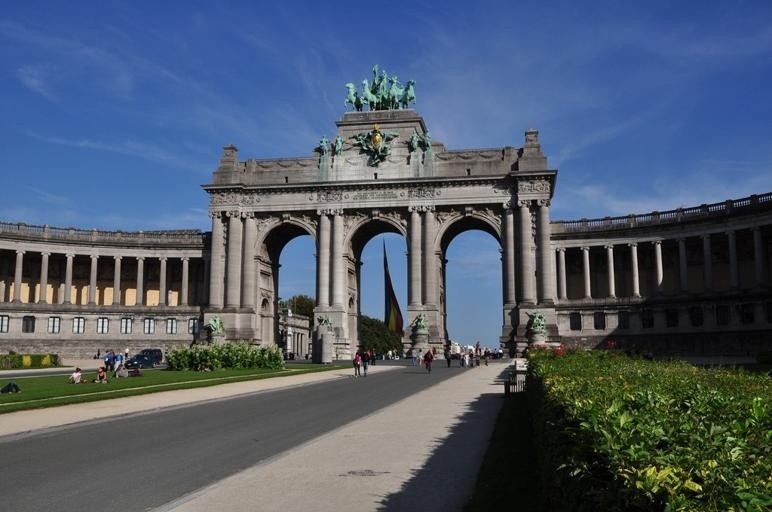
[124,354,160,369]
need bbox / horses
[344,76,416,111]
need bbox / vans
[140,349,162,364]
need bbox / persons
[412,347,436,373]
[444,345,503,368]
[353,345,403,378]
[1,382,22,394]
[104,348,143,378]
[93,367,109,384]
[66,368,87,384]
